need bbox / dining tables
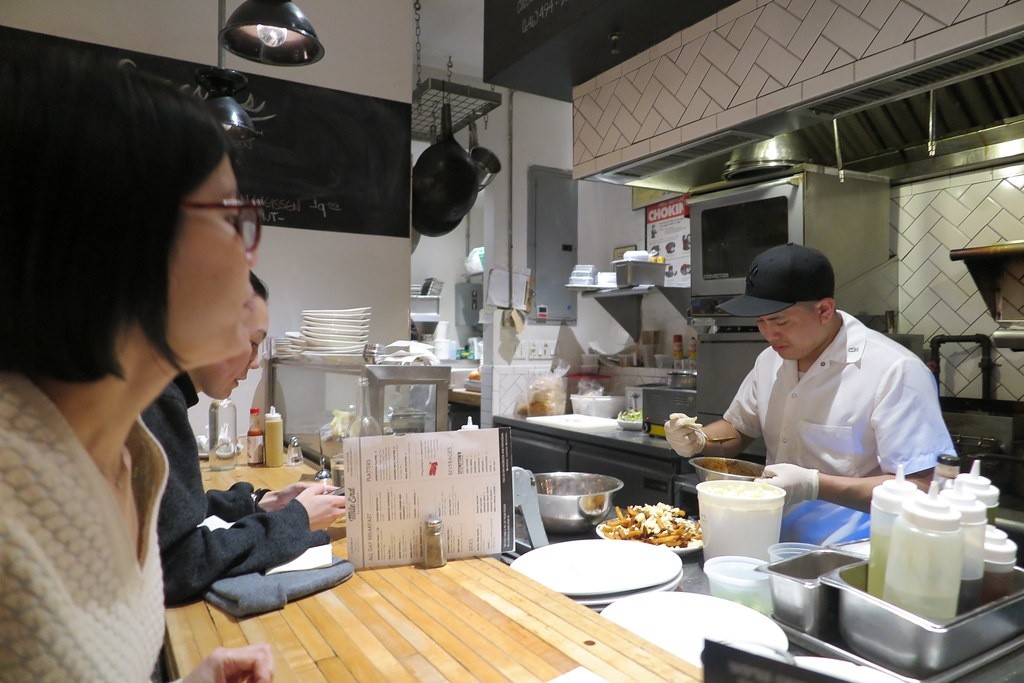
[163,438,704,683]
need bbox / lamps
[196,0,326,139]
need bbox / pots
[413,103,499,236]
[665,371,697,390]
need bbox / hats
[716,241,835,317]
[203,553,355,617]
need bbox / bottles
[867,464,928,601]
[208,394,237,471]
[953,460,1000,526]
[983,525,1018,604]
[688,337,697,359]
[933,454,961,491]
[285,437,303,466]
[264,405,284,467]
[882,481,962,628]
[422,518,447,569]
[246,407,265,465]
[672,334,684,360]
[349,377,382,437]
[460,416,479,430]
[939,478,988,617]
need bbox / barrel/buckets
[695,480,787,561]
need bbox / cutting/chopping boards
[526,414,617,431]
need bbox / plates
[200,445,245,461]
[617,420,642,431]
[507,515,908,682]
[274,304,384,365]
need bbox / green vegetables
[617,408,643,422]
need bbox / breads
[517,390,553,416]
[468,371,481,381]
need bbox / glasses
[182,199,265,251]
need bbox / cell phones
[323,486,345,496]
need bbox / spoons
[665,420,735,443]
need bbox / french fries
[601,506,701,548]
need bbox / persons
[1,55,274,683]
[665,243,958,511]
[139,266,345,607]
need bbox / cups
[766,541,821,562]
[616,344,697,370]
[330,453,345,509]
[702,556,774,617]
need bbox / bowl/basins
[689,457,764,480]
[534,471,625,535]
[722,131,826,180]
